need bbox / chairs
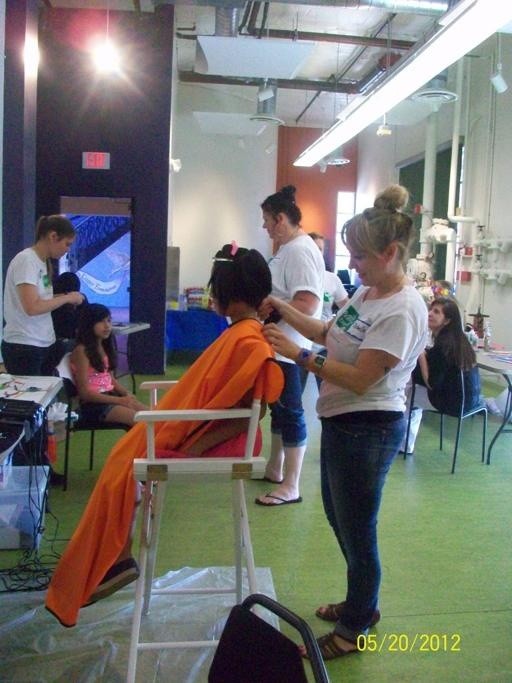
[201,595,333,682]
[62,351,130,492]
[129,355,272,682]
[402,352,512,475]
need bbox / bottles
[467,326,492,353]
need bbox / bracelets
[298,348,312,366]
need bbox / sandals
[295,633,367,662]
[317,601,346,622]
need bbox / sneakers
[89,552,143,603]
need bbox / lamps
[236,0,510,166]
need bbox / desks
[164,307,230,368]
[0,374,68,462]
[426,344,512,465]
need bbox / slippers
[257,489,302,510]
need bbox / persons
[46,240,284,628]
[0,210,149,489]
[261,183,429,659]
[251,185,328,506]
[400,295,480,457]
[308,232,349,388]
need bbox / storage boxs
[0,453,13,491]
[0,467,48,551]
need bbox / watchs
[311,355,325,376]
